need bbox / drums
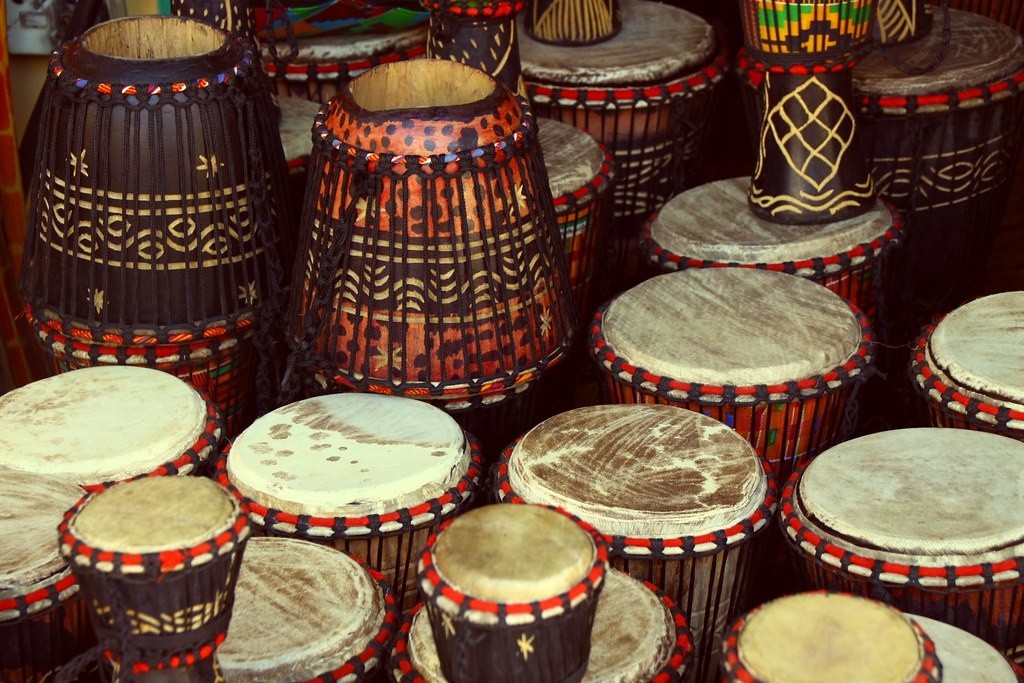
[213,391,486,613]
[24,302,262,447]
[0,471,91,683]
[416,503,610,683]
[527,116,617,326]
[57,473,255,683]
[721,611,1024,683]
[493,403,778,683]
[853,5,1024,323]
[777,427,1024,669]
[422,0,531,109]
[388,562,695,683]
[19,14,290,337]
[738,0,879,225]
[286,57,574,463]
[522,0,623,47]
[258,23,428,104]
[271,97,322,206]
[0,364,224,494]
[212,535,398,683]
[514,0,730,273]
[726,589,943,683]
[641,174,905,335]
[909,290,1024,442]
[589,267,876,490]
